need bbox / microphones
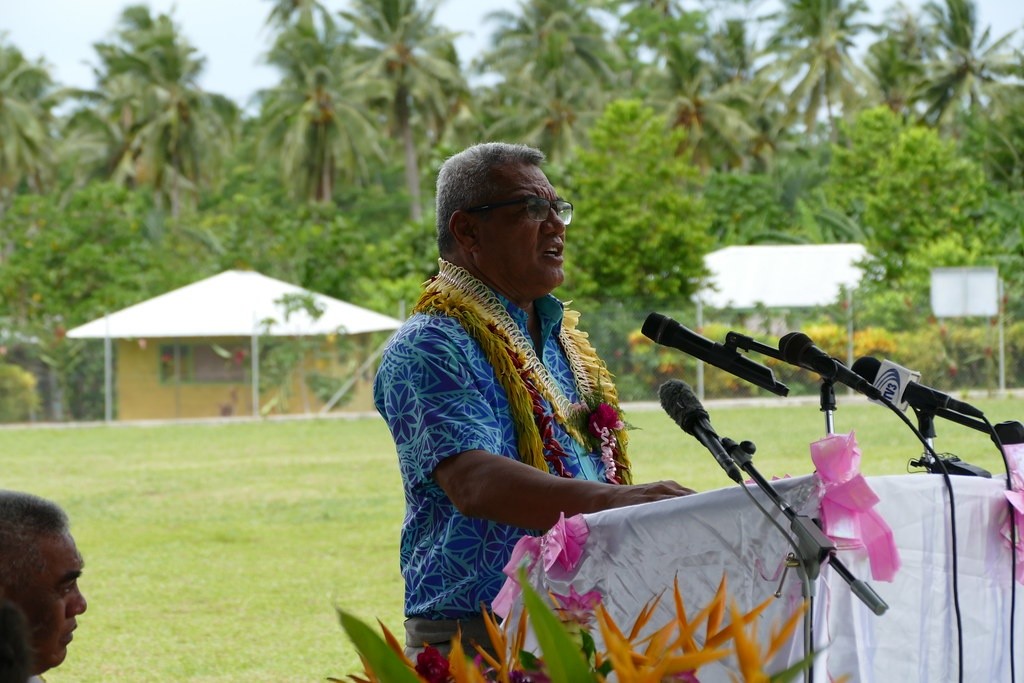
[660,379,743,484]
[849,356,985,419]
[779,331,882,401]
[641,312,790,398]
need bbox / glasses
[475,197,572,226]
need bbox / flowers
[328,559,850,683]
[570,370,641,454]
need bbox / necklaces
[416,255,634,487]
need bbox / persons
[0,489,86,683]
[370,143,701,672]
